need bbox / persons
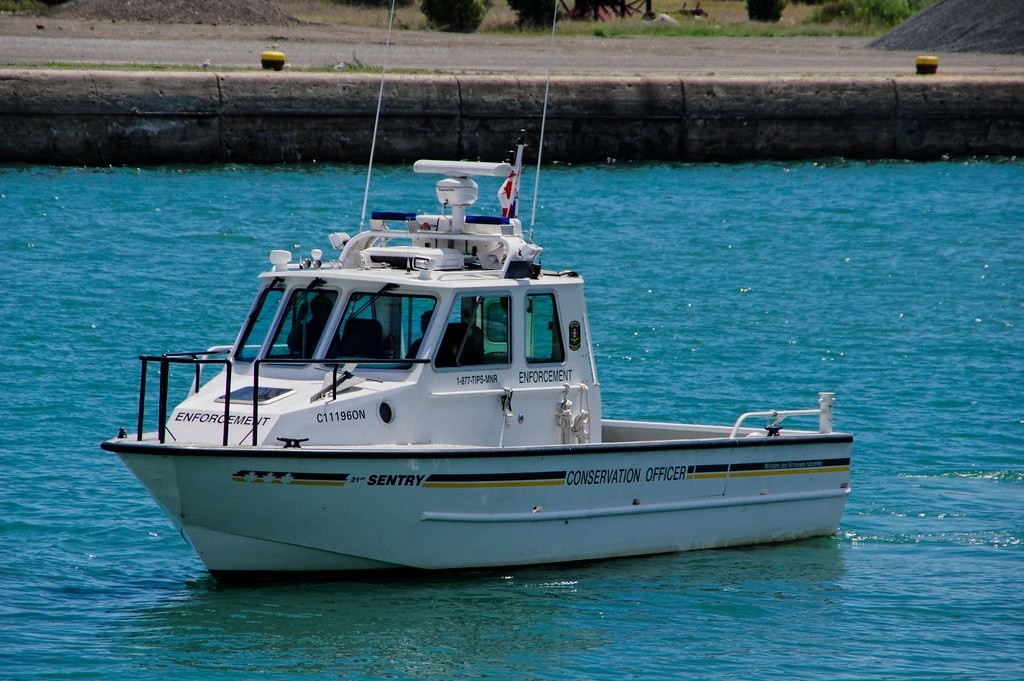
[287,296,340,358]
[404,310,459,367]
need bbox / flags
[498,169,519,218]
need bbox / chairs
[447,323,485,364]
[339,317,382,357]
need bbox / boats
[100,1,854,591]
